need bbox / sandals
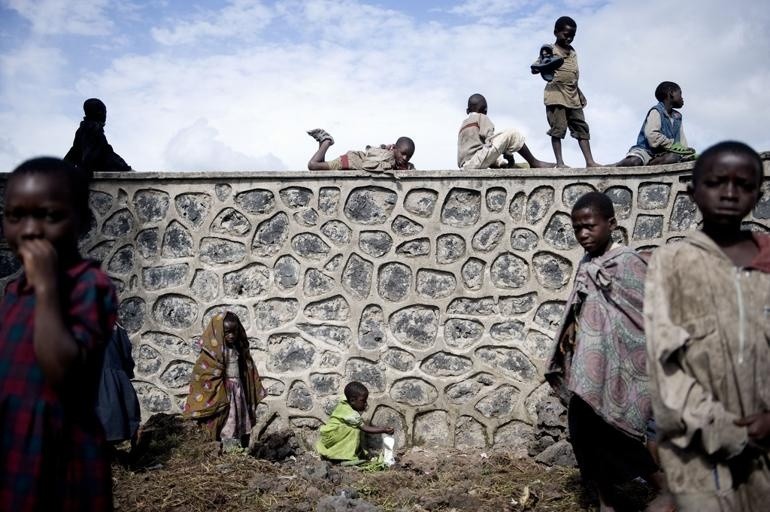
[306,128,334,146]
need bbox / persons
[95,320,141,442]
[306,128,415,174]
[642,141,769,511]
[0,157,119,512]
[457,94,555,169]
[183,310,268,441]
[64,98,131,172]
[531,17,604,166]
[615,81,696,167]
[544,191,649,511]
[312,382,394,462]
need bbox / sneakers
[666,141,696,162]
[531,44,564,82]
